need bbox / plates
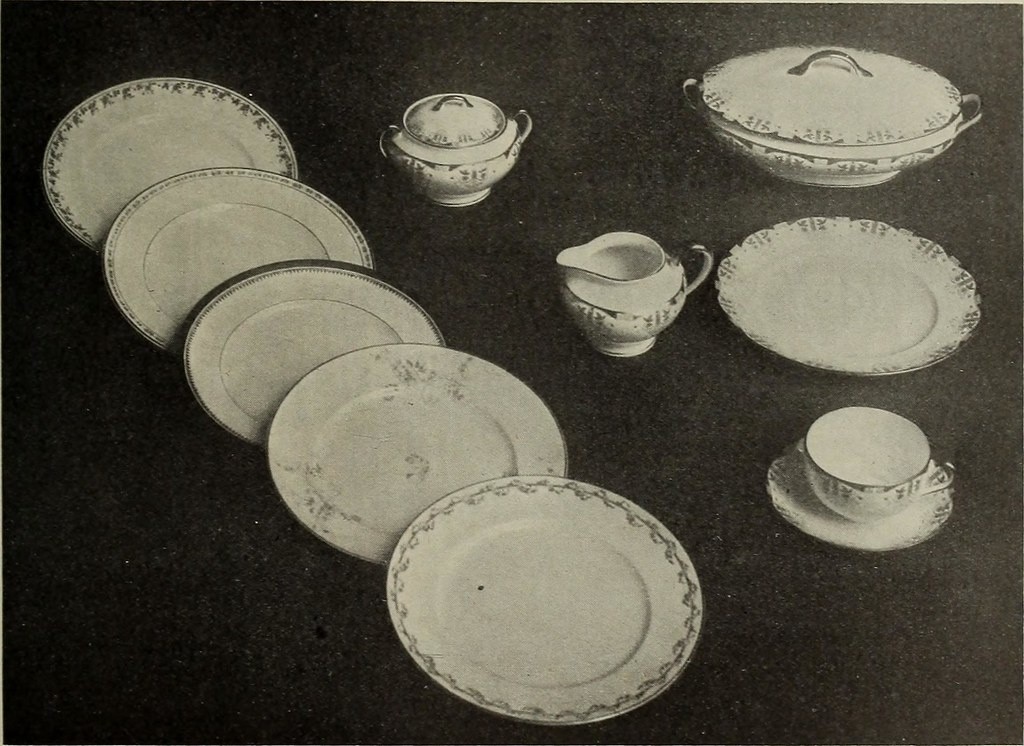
[717,218,981,377]
[174,259,445,450]
[44,77,299,255]
[387,474,703,725]
[102,167,375,353]
[266,342,567,562]
[768,456,955,549]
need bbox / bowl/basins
[683,44,979,187]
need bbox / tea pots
[557,232,713,354]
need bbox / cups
[799,408,955,522]
[380,94,531,208]
